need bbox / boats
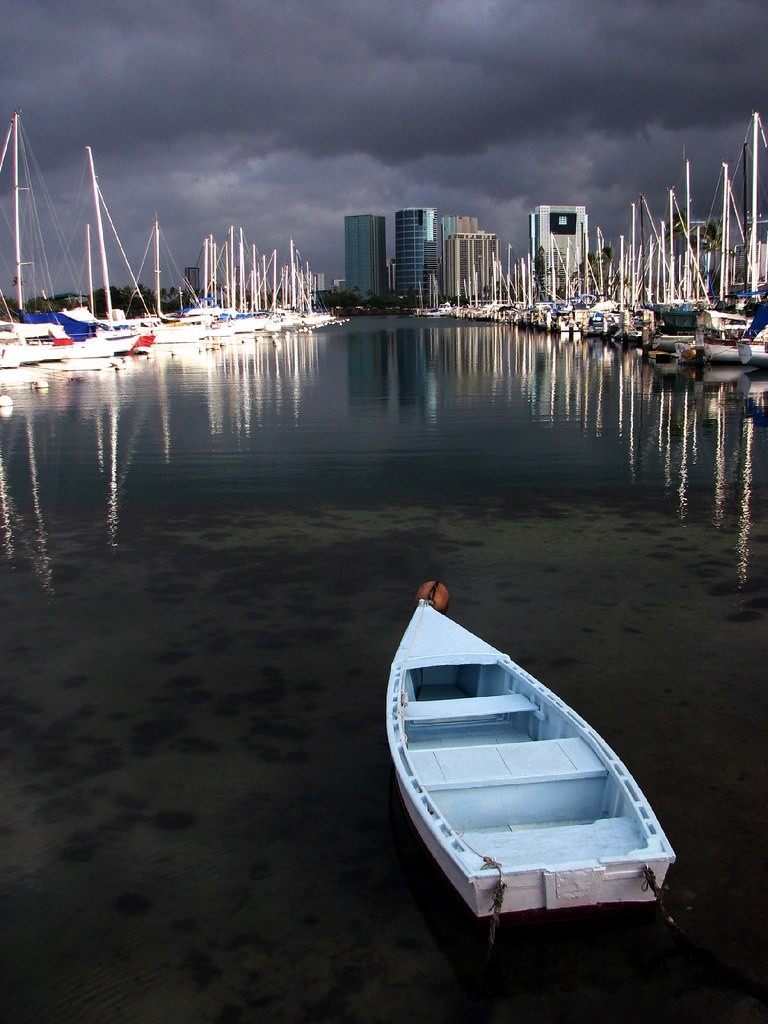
[388,602,677,919]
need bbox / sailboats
[0,111,339,373]
[414,110,768,372]
